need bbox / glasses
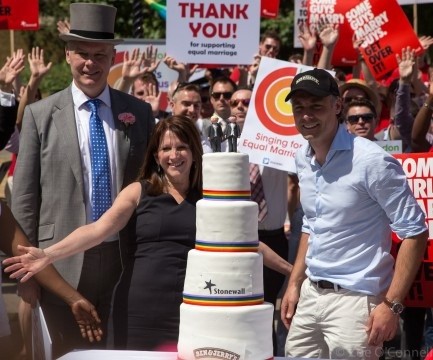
[228,98,251,108]
[266,43,280,52]
[345,114,376,124]
[211,90,233,100]
[342,96,369,103]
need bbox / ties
[86,100,112,221]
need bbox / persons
[1,116,294,352]
[11,39,159,359]
[266,66,429,360]
[331,65,433,154]
[223,114,241,154]
[223,86,291,358]
[112,45,236,153]
[236,66,266,86]
[0,42,52,125]
[206,116,224,153]
[0,199,105,344]
[237,13,432,66]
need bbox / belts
[310,278,341,289]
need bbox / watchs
[380,296,405,316]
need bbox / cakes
[177,114,275,360]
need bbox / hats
[338,76,381,114]
[285,68,339,102]
[58,3,127,45]
